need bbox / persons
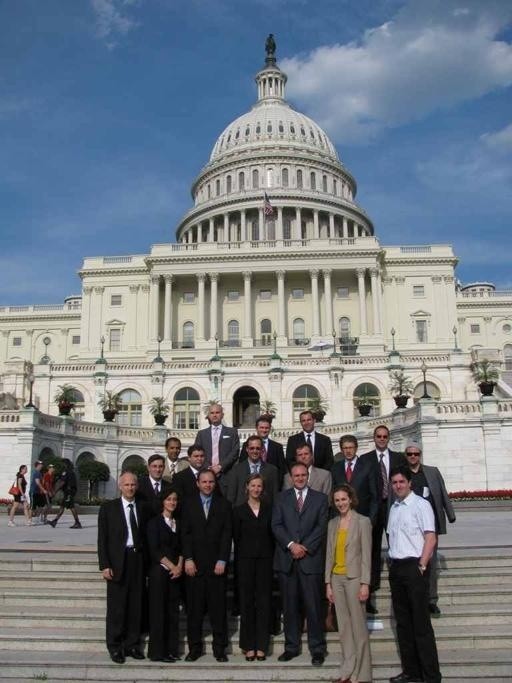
[7,459,81,529]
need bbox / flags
[264,192,274,215]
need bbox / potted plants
[146,394,173,425]
[305,393,330,421]
[97,386,123,422]
[388,366,414,409]
[468,356,504,395]
[54,383,80,416]
[353,387,378,416]
[257,398,277,425]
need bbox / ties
[346,462,352,482]
[128,504,139,546]
[307,434,312,452]
[251,442,267,474]
[213,427,220,477]
[380,454,388,499]
[296,491,303,512]
[155,464,175,496]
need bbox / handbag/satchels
[8,487,20,495]
[325,602,338,632]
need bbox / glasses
[407,453,420,456]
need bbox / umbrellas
[307,339,335,356]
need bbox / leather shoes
[429,605,440,614]
[333,678,352,683]
[246,650,265,661]
[279,651,300,661]
[109,646,202,663]
[366,598,378,614]
[312,656,324,665]
[390,670,425,683]
[214,651,228,662]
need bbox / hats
[405,441,422,452]
